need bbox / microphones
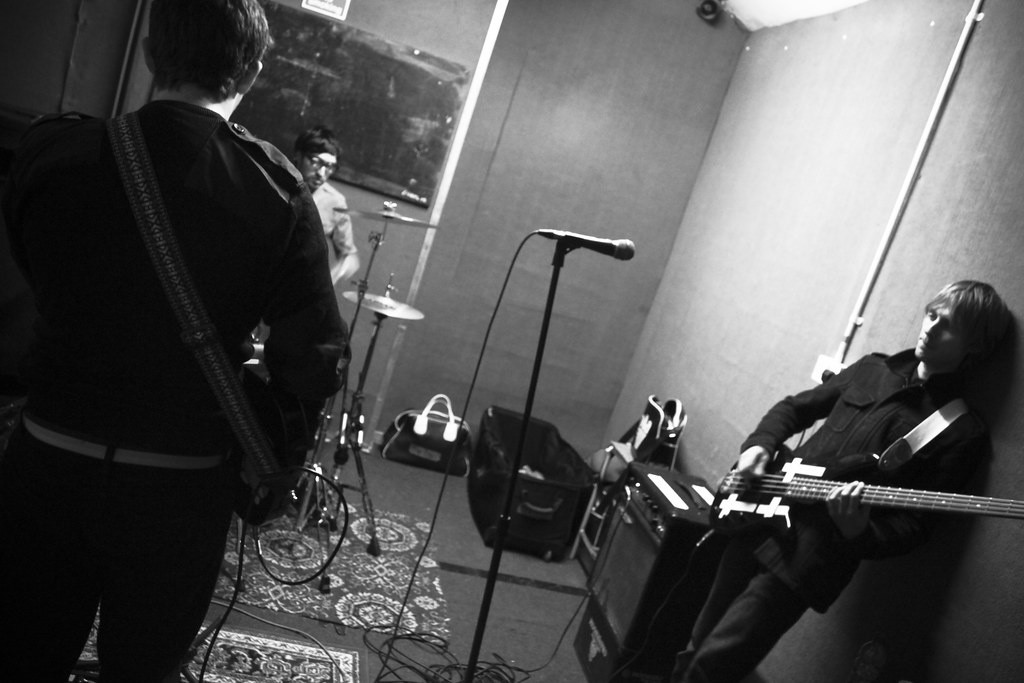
[537,227,636,261]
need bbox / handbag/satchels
[382,393,473,477]
[629,394,688,470]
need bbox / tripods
[285,316,383,595]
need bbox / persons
[0,0,360,683]
[670,281,1010,683]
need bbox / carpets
[68,605,368,682]
[210,488,451,645]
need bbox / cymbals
[330,198,443,231]
[341,290,425,322]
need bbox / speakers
[592,463,717,660]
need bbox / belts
[22,415,222,469]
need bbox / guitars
[225,360,312,530]
[706,440,1024,538]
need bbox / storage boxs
[463,402,594,558]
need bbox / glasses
[304,152,338,175]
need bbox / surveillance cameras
[698,0,722,22]
